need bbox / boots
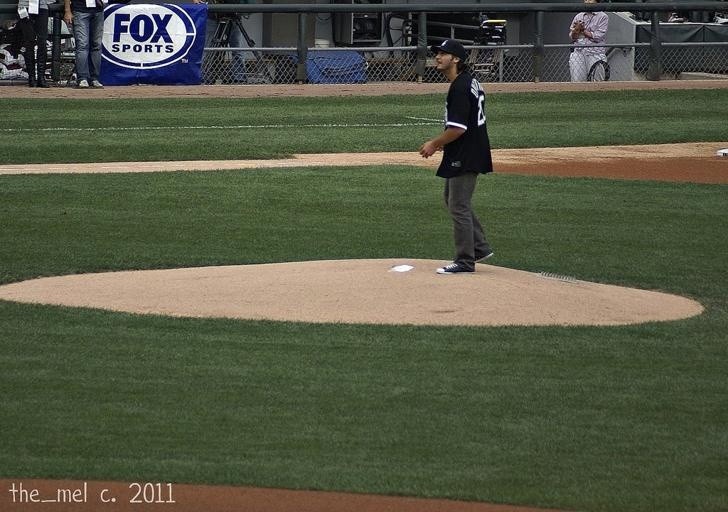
[24,46,50,88]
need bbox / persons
[64,0,109,89]
[418,39,495,274]
[568,0,609,82]
[634,0,728,24]
[17,0,57,89]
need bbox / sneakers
[90,80,103,88]
[475,248,495,262]
[78,79,90,89]
[436,259,475,275]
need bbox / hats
[427,38,467,63]
